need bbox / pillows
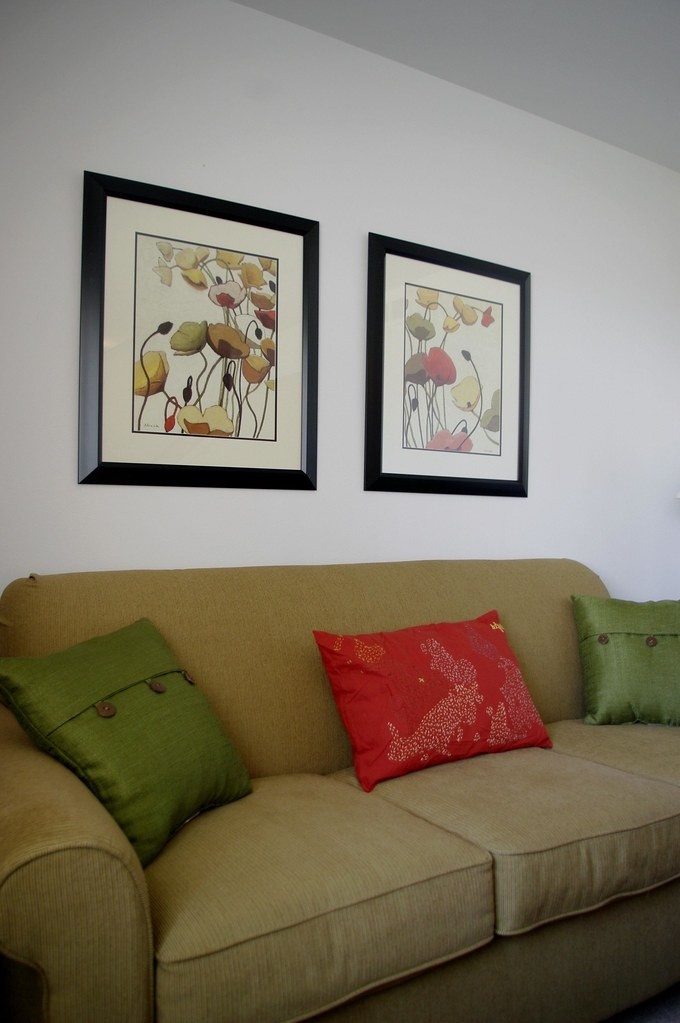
[312,610,553,793]
[571,595,680,727]
[0,617,252,869]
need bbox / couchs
[0,558,680,1023]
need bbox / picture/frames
[363,232,531,500]
[76,169,319,493]
